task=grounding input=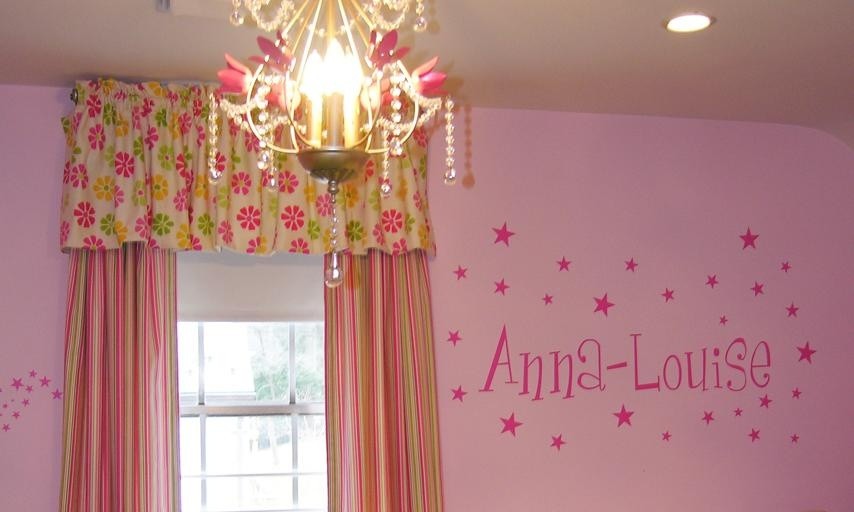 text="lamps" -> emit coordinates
[204,0,459,289]
